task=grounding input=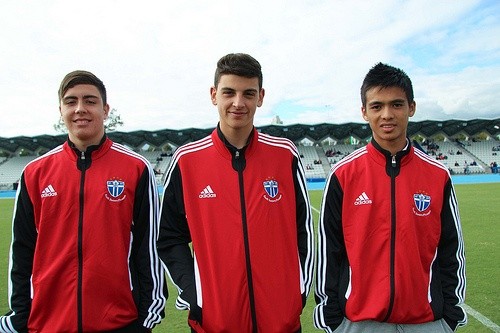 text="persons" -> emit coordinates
[154,141,500,175]
[0,70,169,333]
[156,54,315,333]
[313,62,466,333]
